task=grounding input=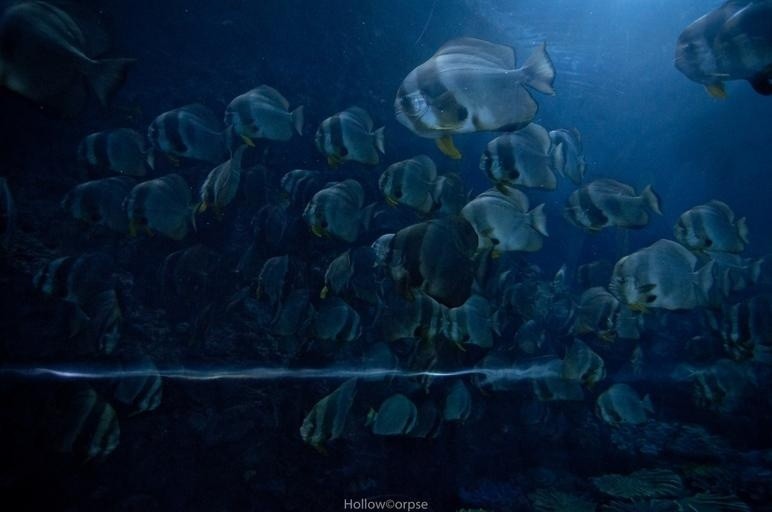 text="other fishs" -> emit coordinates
[31,84,771,458]
